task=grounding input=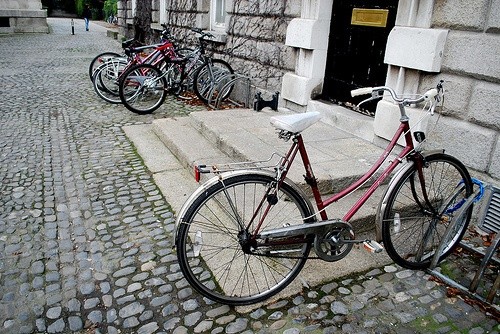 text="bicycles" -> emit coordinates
[119,28,235,115]
[89,23,209,104]
[172,80,474,306]
